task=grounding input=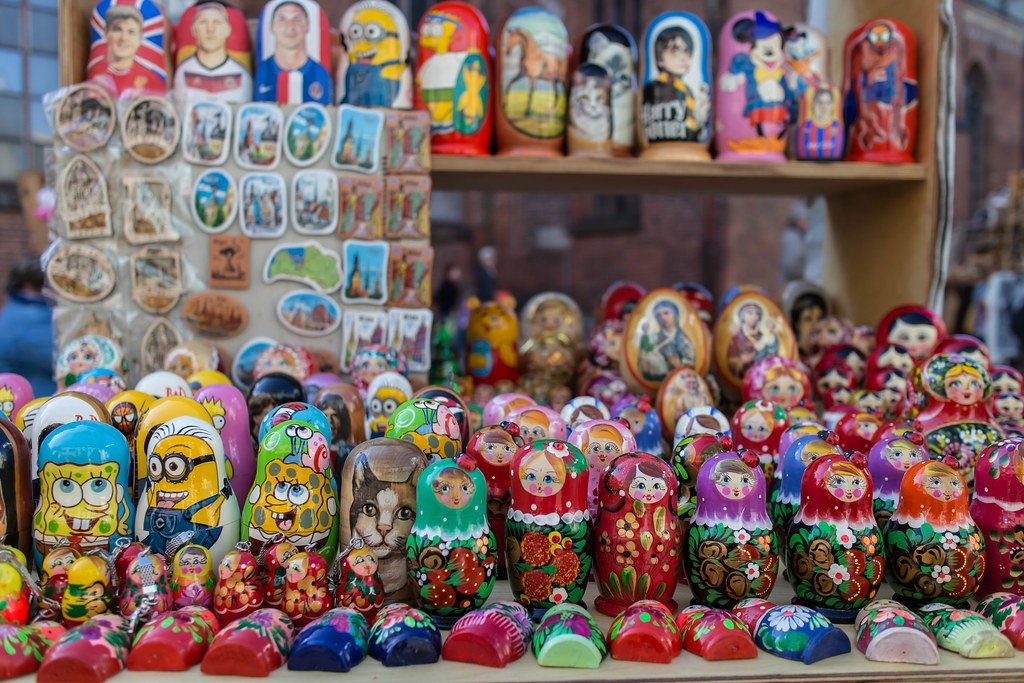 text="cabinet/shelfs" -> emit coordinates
[56,0,955,391]
